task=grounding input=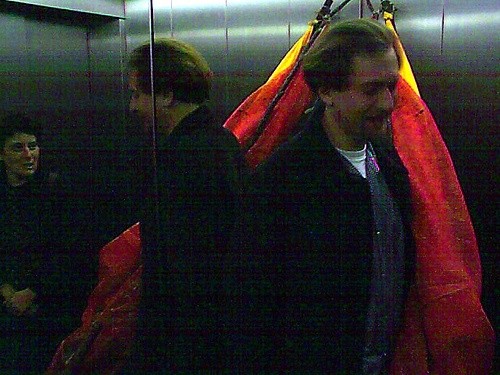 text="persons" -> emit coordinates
[121,40,246,375]
[218,18,416,375]
[0,115,81,375]
